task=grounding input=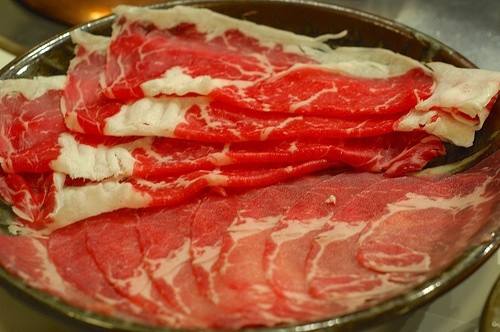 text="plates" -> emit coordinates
[0,0,500,332]
